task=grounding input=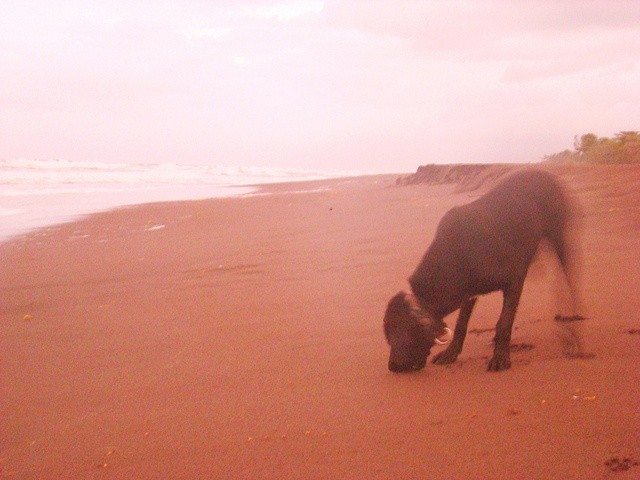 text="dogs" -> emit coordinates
[380,163,592,375]
